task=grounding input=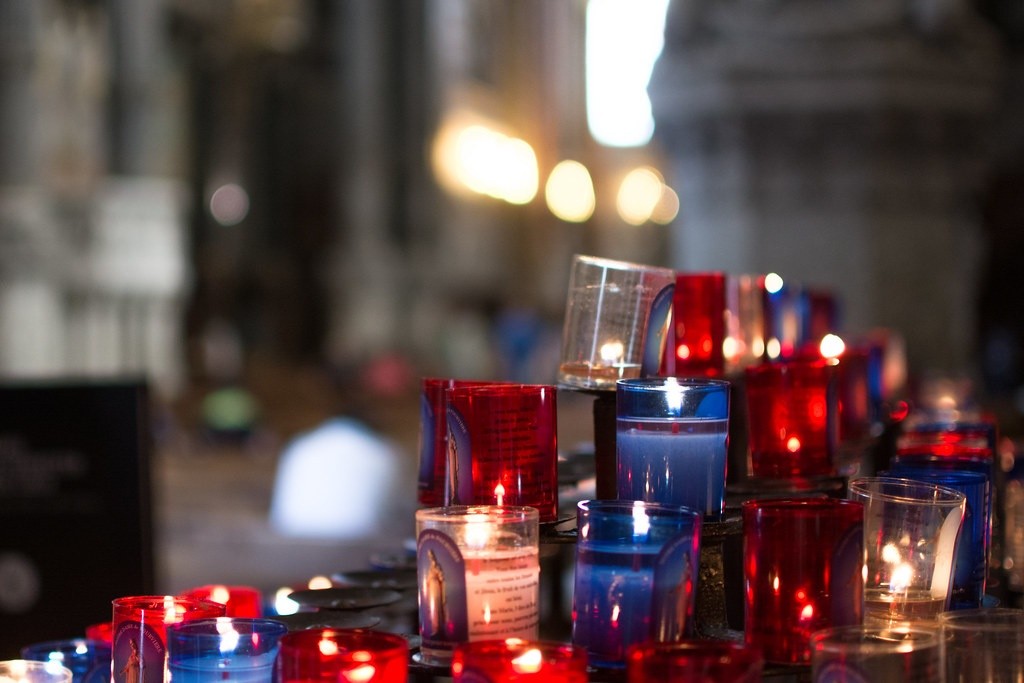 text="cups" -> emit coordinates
[0,254,1024,683]
